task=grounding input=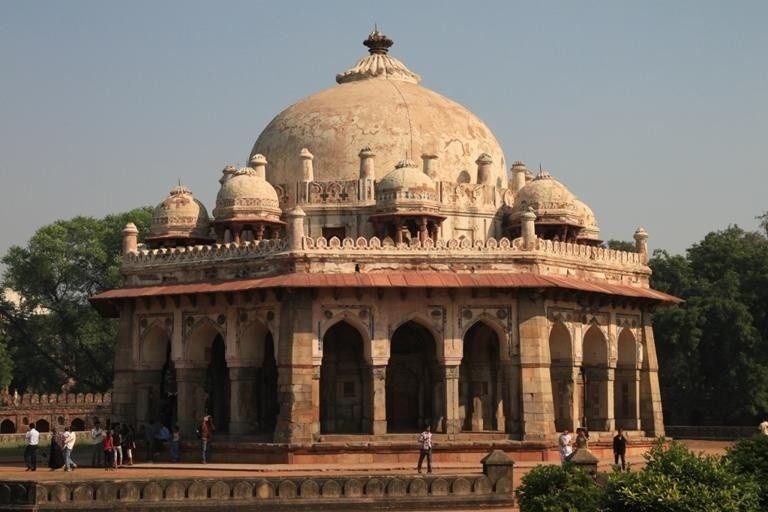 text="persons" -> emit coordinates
[756,416,768,438]
[611,428,627,472]
[197,413,216,465]
[23,423,39,471]
[574,429,589,450]
[417,425,433,474]
[45,419,184,473]
[558,428,573,468]
[574,427,581,449]
